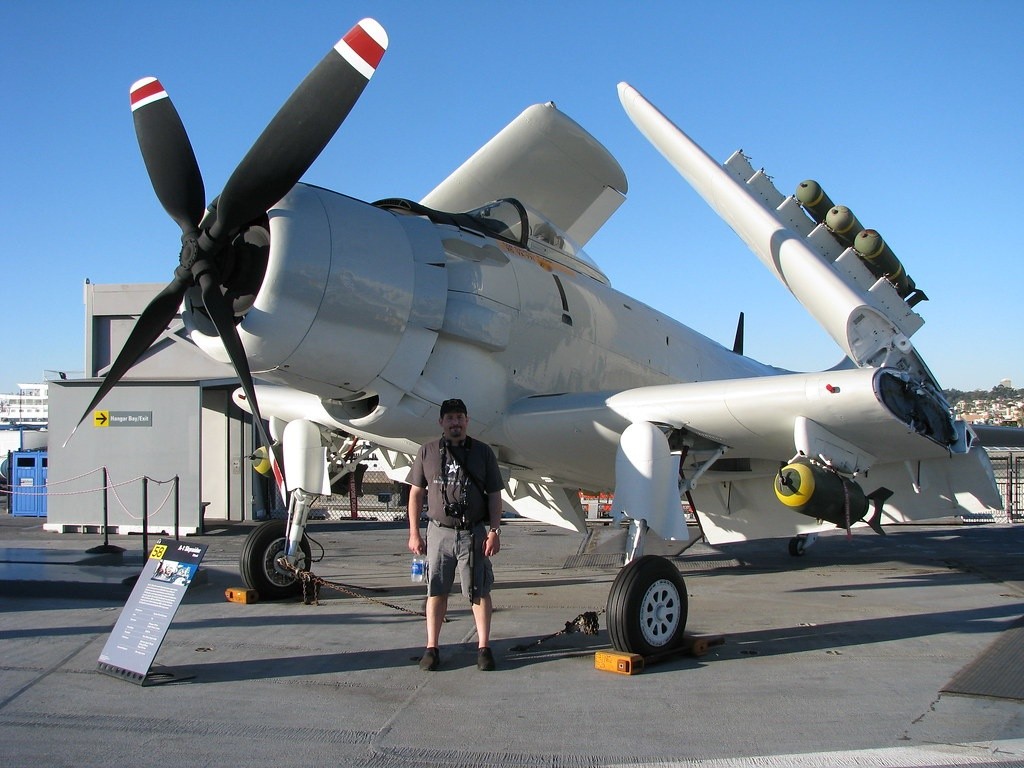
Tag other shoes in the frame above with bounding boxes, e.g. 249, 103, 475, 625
478, 646, 494, 671
419, 647, 440, 671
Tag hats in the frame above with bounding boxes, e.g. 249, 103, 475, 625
440, 398, 468, 418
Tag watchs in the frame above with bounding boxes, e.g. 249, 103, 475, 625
489, 527, 501, 535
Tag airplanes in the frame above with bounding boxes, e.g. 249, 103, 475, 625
59, 15, 1005, 660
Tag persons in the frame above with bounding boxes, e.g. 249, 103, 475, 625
404, 398, 504, 670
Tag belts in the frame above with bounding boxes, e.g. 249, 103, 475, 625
430, 518, 471, 530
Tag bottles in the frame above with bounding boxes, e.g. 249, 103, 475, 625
411, 555, 424, 582
